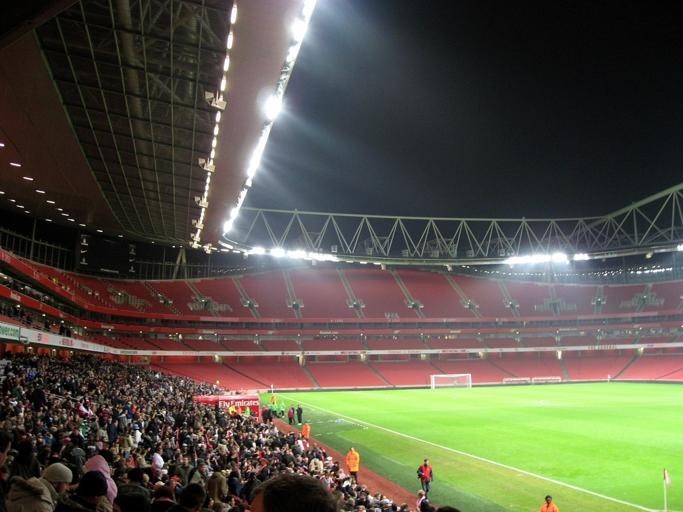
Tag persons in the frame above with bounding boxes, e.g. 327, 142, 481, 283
539, 495, 560, 512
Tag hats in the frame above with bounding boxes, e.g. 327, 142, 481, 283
76, 470, 108, 496
43, 462, 73, 483
